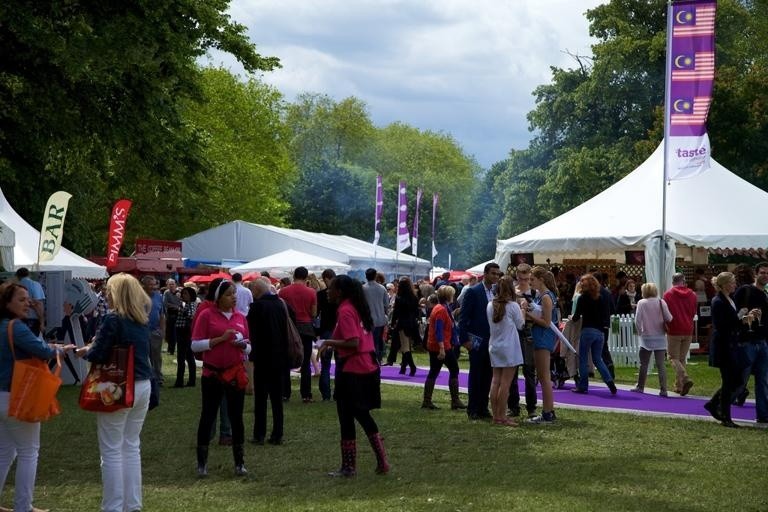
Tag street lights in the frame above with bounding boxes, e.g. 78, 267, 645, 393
278, 296, 305, 370
78, 313, 136, 416
8, 320, 63, 423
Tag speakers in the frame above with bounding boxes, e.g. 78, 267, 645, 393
448, 378, 468, 412
399, 351, 408, 374
366, 431, 391, 475
420, 378, 441, 413
228, 441, 248, 477
404, 350, 416, 376
195, 444, 212, 477
326, 438, 359, 480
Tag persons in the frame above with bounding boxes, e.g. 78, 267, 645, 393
74, 254, 768, 510
1, 282, 79, 510
15, 268, 47, 338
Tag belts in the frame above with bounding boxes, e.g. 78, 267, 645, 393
747, 308, 764, 332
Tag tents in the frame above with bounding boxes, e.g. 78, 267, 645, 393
0, 187, 107, 388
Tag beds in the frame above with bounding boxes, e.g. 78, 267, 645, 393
227, 247, 353, 275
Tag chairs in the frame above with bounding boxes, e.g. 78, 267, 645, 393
704, 387, 767, 427
571, 378, 698, 399
466, 405, 559, 427
